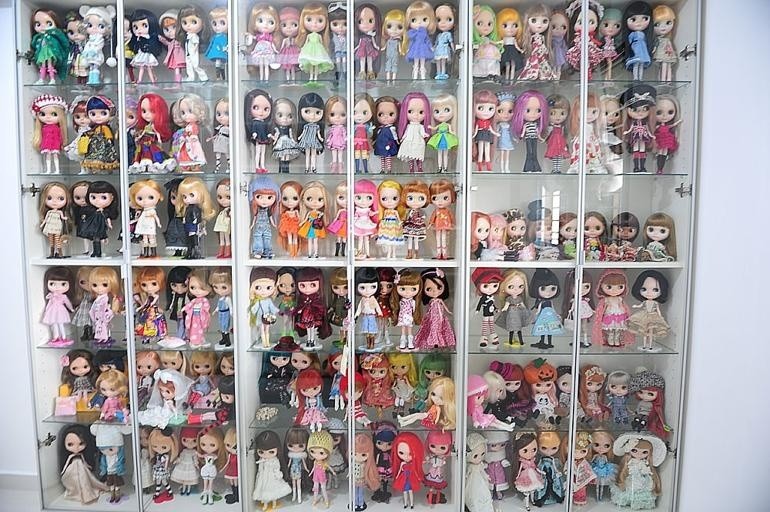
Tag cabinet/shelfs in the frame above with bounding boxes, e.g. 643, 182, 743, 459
18, 0, 700, 511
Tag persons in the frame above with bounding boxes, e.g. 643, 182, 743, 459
208, 266, 236, 349
193, 427, 227, 506
176, 4, 210, 83
148, 427, 180, 496
187, 375, 235, 437
126, 8, 163, 85
397, 91, 431, 175
136, 367, 197, 429
60, 8, 90, 84
508, 428, 547, 512
481, 431, 511, 501
133, 428, 154, 495
353, 179, 378, 258
259, 350, 294, 410
77, 4, 116, 83
611, 429, 668, 508
67, 181, 97, 257
424, 92, 461, 173
621, 0, 654, 82
338, 370, 376, 428
559, 428, 597, 506
431, 3, 458, 81
482, 370, 528, 429
210, 349, 235, 375
353, 92, 376, 173
268, 98, 301, 172
491, 91, 517, 174
391, 431, 426, 510
553, 365, 594, 425
473, 89, 501, 170
511, 89, 546, 172
375, 8, 406, 81
502, 209, 559, 262
37, 182, 72, 258
245, 89, 275, 172
171, 427, 200, 496
305, 430, 338, 510
252, 431, 294, 511
628, 370, 672, 439
348, 432, 380, 508
390, 269, 424, 350
32, 94, 70, 176
59, 424, 109, 502
561, 268, 598, 349
358, 354, 396, 419
91, 422, 132, 504
618, 83, 658, 173
283, 427, 314, 503
593, 8, 622, 81
249, 176, 282, 258
129, 92, 177, 172
205, 96, 231, 174
212, 177, 230, 255
275, 264, 298, 344
129, 181, 166, 259
326, 429, 350, 492
293, 268, 334, 347
40, 266, 75, 346
484, 214, 537, 262
495, 5, 527, 82
387, 351, 418, 416
594, 94, 626, 176
398, 179, 432, 258
86, 181, 120, 258
583, 213, 633, 262
375, 180, 404, 258
326, 349, 344, 411
284, 349, 321, 408
625, 269, 673, 351
602, 369, 635, 425
584, 427, 616, 501
650, 4, 677, 81
179, 349, 219, 409
176, 92, 212, 172
163, 178, 190, 257
465, 433, 497, 512
116, 183, 142, 255
593, 269, 636, 346
290, 369, 329, 431
495, 268, 532, 345
566, 0, 604, 81
640, 212, 678, 262
325, 181, 348, 256
323, 95, 347, 172
557, 211, 600, 261
133, 264, 168, 346
165, 266, 193, 341
155, 350, 192, 377
81, 94, 119, 174
298, 180, 332, 258
396, 375, 457, 434
246, 4, 281, 83
60, 349, 95, 408
157, 8, 186, 83
544, 8, 571, 79
372, 95, 400, 174
181, 268, 216, 349
471, 212, 520, 262
293, 4, 336, 82
401, 1, 438, 81
93, 369, 129, 422
27, 8, 74, 84
135, 351, 160, 409
414, 268, 456, 349
528, 268, 568, 349
112, 14, 138, 82
114, 95, 141, 172
274, 7, 301, 81
539, 93, 571, 174
578, 364, 612, 422
87, 266, 123, 347
533, 428, 568, 506
354, 266, 384, 349
323, 1, 348, 81
427, 178, 456, 258
86, 350, 127, 409
473, 267, 506, 346
407, 352, 449, 415
372, 422, 400, 503
521, 1, 557, 80
295, 92, 325, 174
326, 266, 351, 345
168, 97, 185, 171
275, 181, 307, 259
217, 428, 238, 503
203, 5, 231, 81
610, 212, 653, 262
377, 267, 398, 346
249, 268, 278, 347
354, 3, 382, 79
568, 92, 606, 174
523, 358, 563, 425
490, 360, 542, 420
419, 431, 453, 506
471, 5, 504, 83
61, 95, 95, 174
647, 94, 683, 174
467, 375, 516, 432
70, 266, 96, 342
527, 201, 570, 260
172, 175, 218, 257
